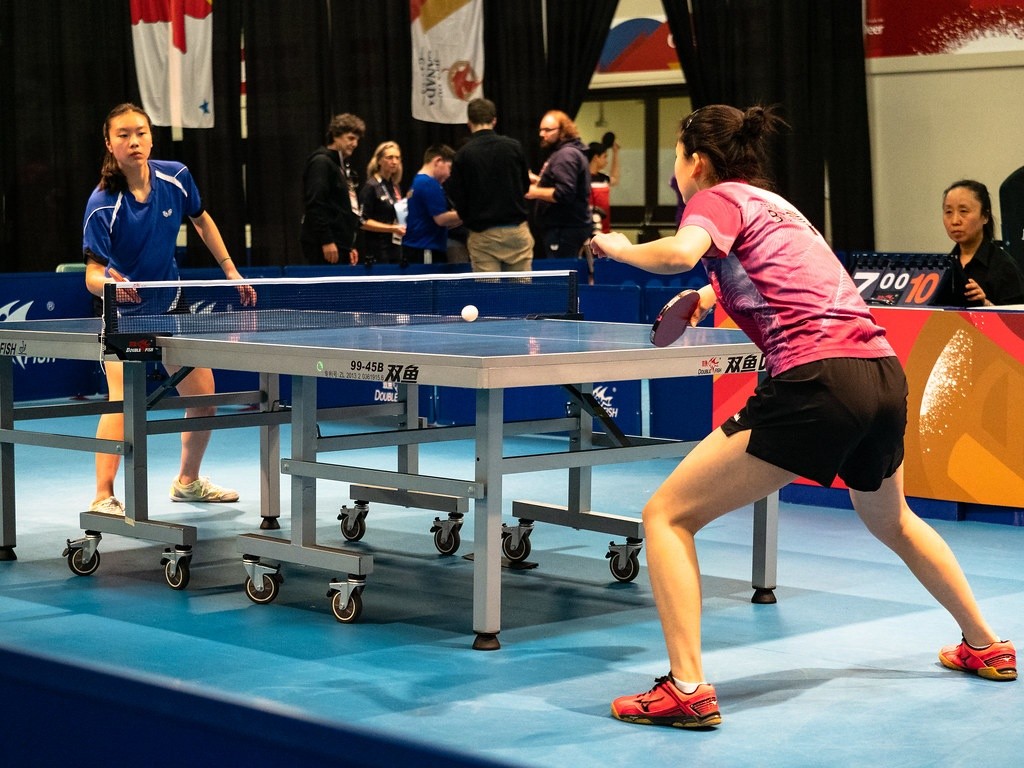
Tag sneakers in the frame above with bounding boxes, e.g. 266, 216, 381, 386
90, 496, 125, 516
938, 631, 1018, 679
170, 476, 240, 502
610, 671, 722, 727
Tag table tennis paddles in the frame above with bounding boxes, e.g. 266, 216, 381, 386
649, 288, 716, 348
108, 268, 141, 303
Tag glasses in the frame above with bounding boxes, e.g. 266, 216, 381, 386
538, 127, 559, 133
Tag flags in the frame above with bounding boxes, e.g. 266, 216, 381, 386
130, 0, 215, 129
409, 0, 485, 124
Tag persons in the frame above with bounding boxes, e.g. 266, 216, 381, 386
940, 179, 1023, 309
81, 103, 259, 521
584, 139, 621, 234
525, 110, 592, 260
445, 97, 535, 284
584, 102, 1018, 730
356, 139, 410, 265
298, 113, 366, 266
400, 142, 464, 264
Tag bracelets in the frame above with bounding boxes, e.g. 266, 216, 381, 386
219, 255, 232, 267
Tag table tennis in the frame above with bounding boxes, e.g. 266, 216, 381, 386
461, 304, 479, 323
